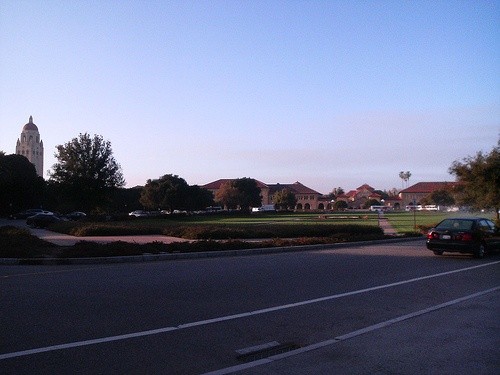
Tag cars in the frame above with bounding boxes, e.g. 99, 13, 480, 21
7, 205, 255, 221
370, 204, 488, 216
426, 217, 500, 259
